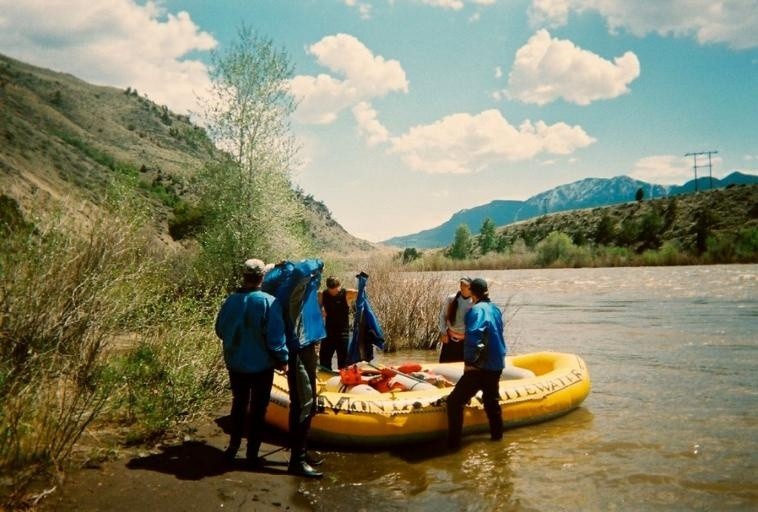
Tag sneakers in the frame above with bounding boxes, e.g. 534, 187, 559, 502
286, 460, 325, 478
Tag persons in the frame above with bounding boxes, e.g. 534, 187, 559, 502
435, 278, 508, 449
317, 270, 369, 374
262, 257, 330, 479
437, 276, 473, 363
212, 257, 290, 471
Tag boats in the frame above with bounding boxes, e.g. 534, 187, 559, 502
243, 347, 592, 451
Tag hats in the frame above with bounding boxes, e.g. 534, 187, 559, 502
241, 258, 265, 279
467, 277, 488, 291
458, 275, 472, 285
